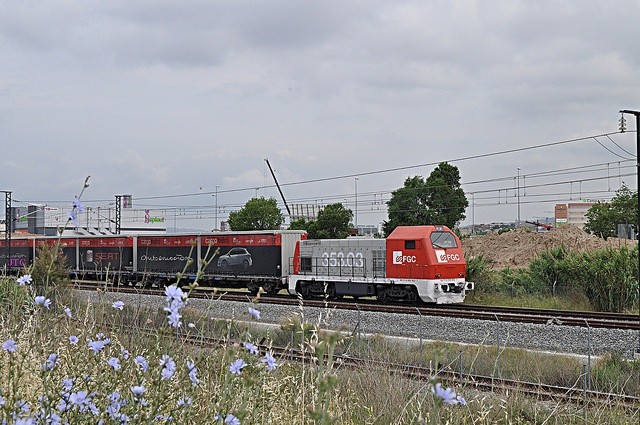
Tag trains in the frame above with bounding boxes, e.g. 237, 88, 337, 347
0, 225, 467, 306
